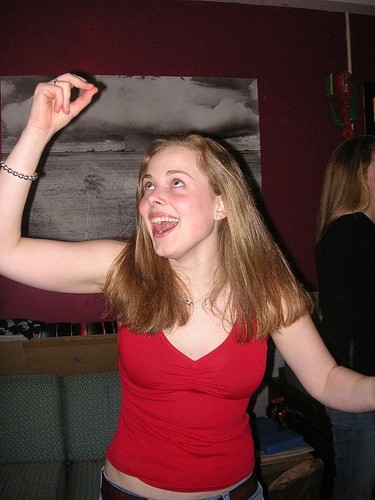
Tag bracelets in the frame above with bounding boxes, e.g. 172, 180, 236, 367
1, 159, 38, 182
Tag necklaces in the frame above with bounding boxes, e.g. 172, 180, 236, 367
183, 293, 208, 306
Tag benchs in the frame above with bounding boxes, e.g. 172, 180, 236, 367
0, 333, 122, 500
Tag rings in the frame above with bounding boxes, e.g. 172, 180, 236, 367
50, 79, 58, 85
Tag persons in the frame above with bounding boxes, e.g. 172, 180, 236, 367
0, 72, 375, 500
314, 136, 375, 500
